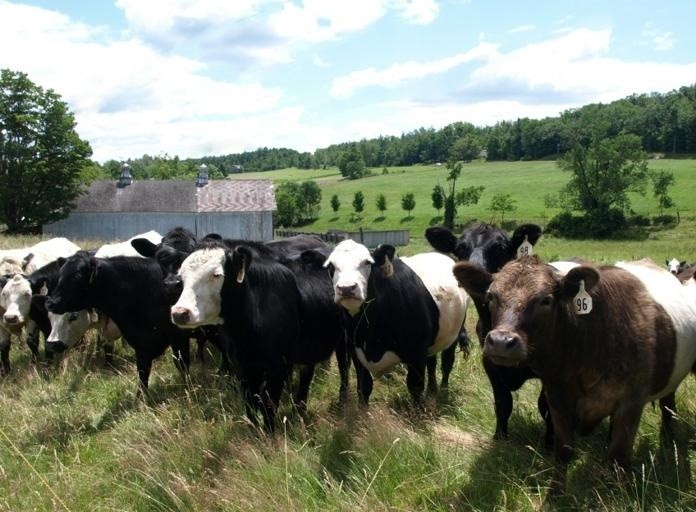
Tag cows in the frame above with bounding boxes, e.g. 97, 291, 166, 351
0, 220, 694, 478
